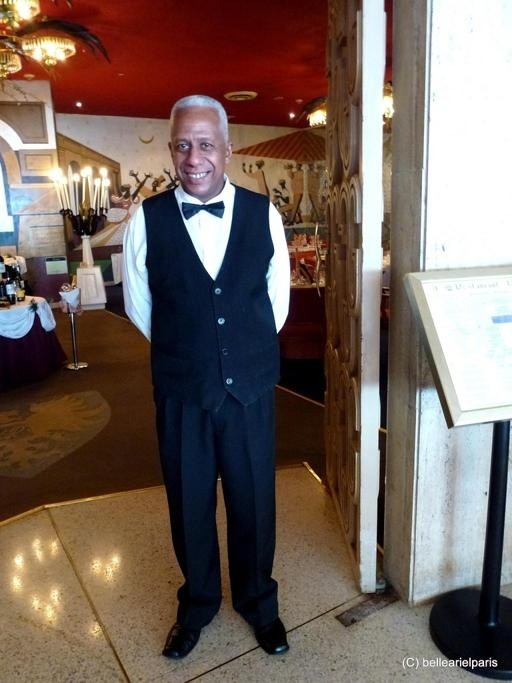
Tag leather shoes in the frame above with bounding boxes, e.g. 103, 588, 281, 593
163, 621, 205, 658
253, 616, 289, 656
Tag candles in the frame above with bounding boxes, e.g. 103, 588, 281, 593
50, 162, 112, 216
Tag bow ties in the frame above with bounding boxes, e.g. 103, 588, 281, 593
182, 200, 226, 220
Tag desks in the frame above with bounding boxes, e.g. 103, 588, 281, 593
0, 293, 67, 386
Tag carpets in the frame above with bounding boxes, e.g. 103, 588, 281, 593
0, 305, 386, 556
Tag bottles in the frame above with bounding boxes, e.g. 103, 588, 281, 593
6, 264, 25, 304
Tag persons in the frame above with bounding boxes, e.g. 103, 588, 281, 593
122, 94, 291, 659
